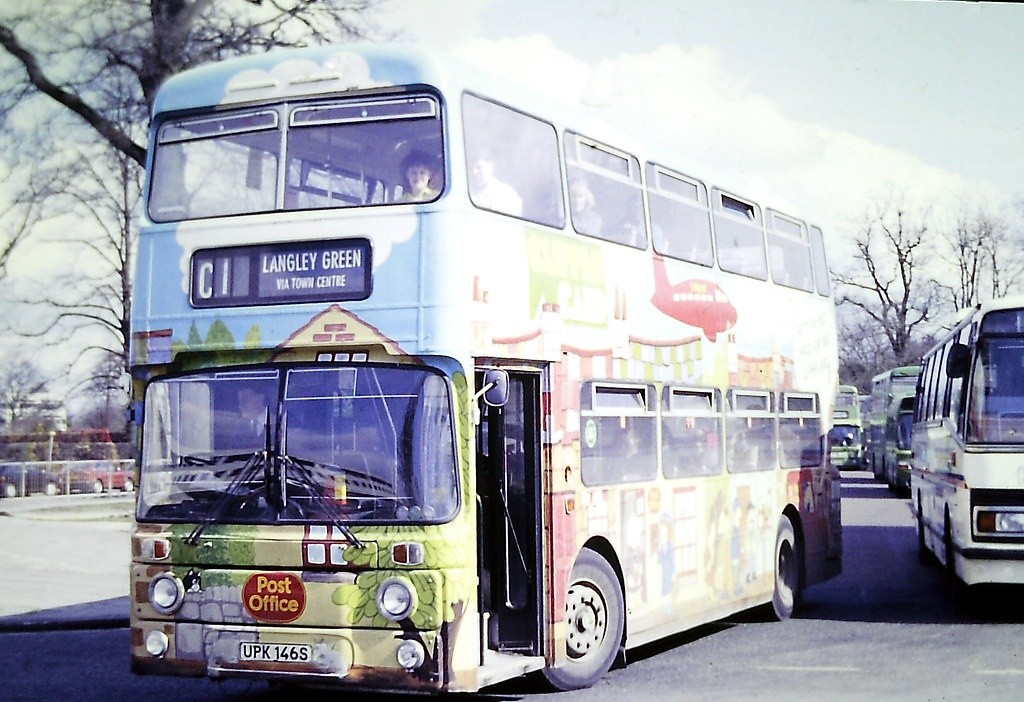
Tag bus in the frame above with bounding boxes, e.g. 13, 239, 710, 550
116, 37, 845, 695
907, 295, 1023, 599
867, 366, 930, 495
828, 385, 873, 471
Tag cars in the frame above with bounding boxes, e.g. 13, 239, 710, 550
60, 461, 136, 494
0, 464, 61, 498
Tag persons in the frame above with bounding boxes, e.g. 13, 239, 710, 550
398, 149, 665, 255
238, 387, 307, 466
611, 403, 817, 480
